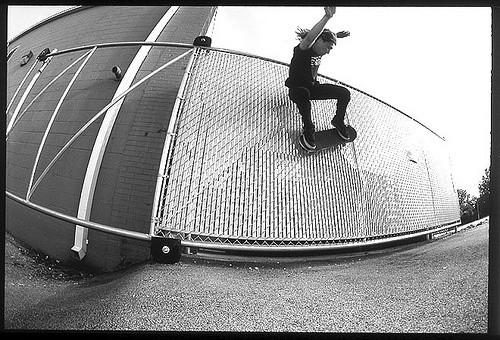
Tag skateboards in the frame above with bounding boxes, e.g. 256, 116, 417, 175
299, 125, 357, 153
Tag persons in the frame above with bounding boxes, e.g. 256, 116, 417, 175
285, 6, 351, 150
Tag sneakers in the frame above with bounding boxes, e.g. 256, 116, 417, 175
303, 121, 316, 150
331, 116, 350, 140
